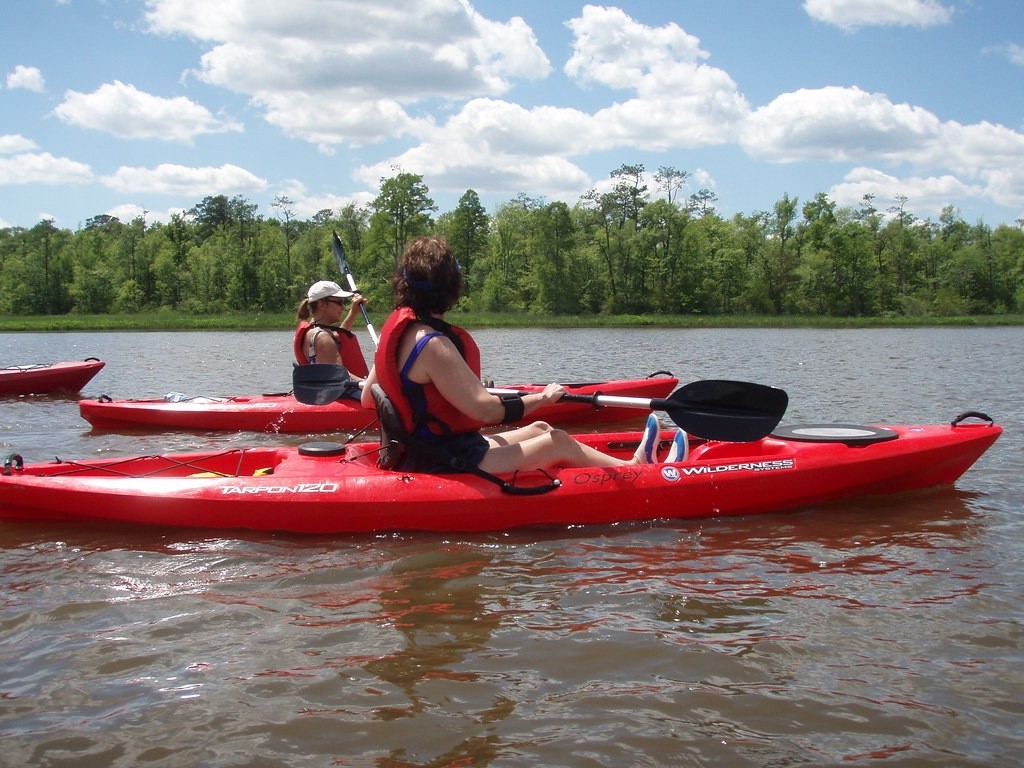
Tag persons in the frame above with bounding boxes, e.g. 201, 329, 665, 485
358, 235, 690, 473
294, 280, 371, 390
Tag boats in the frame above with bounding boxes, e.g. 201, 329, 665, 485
0, 357, 106, 397
0, 411, 1007, 539
75, 371, 682, 434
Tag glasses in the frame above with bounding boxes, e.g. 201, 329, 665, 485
323, 299, 343, 306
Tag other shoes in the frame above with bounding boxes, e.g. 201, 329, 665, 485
634, 413, 660, 464
487, 380, 494, 388
663, 427, 689, 463
482, 380, 488, 387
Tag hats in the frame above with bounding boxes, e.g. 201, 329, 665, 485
306, 280, 354, 303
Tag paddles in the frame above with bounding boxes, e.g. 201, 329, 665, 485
483, 379, 788, 443
331, 229, 378, 346
